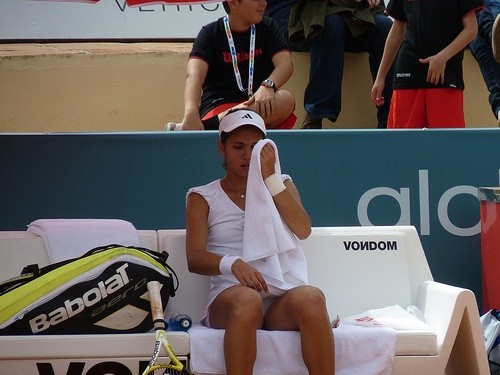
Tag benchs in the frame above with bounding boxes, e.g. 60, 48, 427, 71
0, 225, 491, 375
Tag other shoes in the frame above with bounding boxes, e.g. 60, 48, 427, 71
300, 113, 323, 129
164, 121, 182, 131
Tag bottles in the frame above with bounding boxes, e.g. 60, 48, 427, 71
168, 312, 192, 332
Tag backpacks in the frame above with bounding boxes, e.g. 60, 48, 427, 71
480, 309, 500, 375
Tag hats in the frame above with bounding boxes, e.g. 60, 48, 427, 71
218, 110, 267, 138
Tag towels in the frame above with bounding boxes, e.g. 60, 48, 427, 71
28, 218, 140, 265
242, 138, 309, 289
188, 322, 397, 374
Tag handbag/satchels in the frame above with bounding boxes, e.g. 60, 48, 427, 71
0, 244, 178, 336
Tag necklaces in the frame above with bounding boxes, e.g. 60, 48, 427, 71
224, 177, 246, 198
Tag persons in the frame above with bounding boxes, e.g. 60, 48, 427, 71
264, 0, 500, 129
166, 0, 295, 130
185, 108, 335, 375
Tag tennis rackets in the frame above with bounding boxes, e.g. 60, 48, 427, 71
142, 281, 192, 375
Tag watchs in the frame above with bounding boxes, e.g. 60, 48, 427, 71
263, 80, 277, 91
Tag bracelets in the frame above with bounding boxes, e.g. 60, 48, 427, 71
265, 173, 287, 197
261, 83, 269, 88
219, 255, 244, 276
